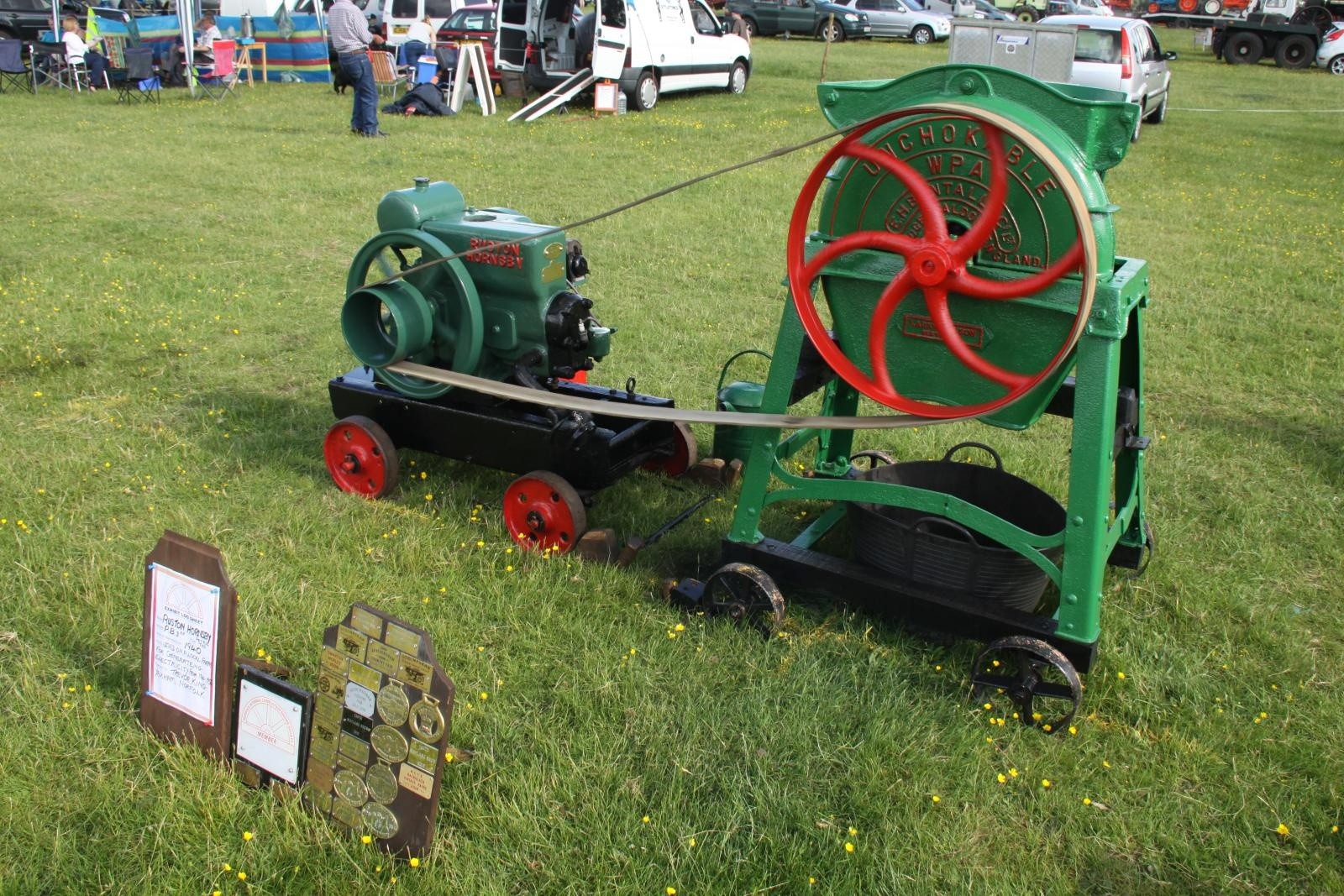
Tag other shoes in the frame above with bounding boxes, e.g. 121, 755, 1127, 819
362, 131, 390, 138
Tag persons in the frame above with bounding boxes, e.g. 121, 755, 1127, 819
730, 7, 753, 47
328, 0, 391, 138
575, 5, 596, 68
367, 13, 382, 36
30, 16, 65, 85
62, 14, 114, 93
404, 14, 437, 76
154, 14, 222, 88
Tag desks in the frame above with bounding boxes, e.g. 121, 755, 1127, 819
237, 41, 266, 82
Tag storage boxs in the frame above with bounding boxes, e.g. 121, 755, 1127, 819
417, 55, 439, 83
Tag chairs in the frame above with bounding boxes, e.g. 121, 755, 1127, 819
1, 31, 253, 106
365, 50, 415, 98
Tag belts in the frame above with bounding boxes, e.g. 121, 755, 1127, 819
341, 48, 365, 56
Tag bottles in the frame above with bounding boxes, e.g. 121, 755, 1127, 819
227, 25, 235, 39
242, 13, 255, 39
617, 88, 626, 115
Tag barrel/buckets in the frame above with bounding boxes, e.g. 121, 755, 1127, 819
849, 442, 1066, 615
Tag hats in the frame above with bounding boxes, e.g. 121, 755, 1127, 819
367, 13, 377, 20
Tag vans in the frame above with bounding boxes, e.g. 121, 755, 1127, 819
493, 0, 755, 109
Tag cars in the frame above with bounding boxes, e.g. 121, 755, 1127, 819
1316, 22, 1344, 75
289, 0, 509, 98
827, 0, 953, 45
936, 0, 1115, 27
1032, 13, 1179, 143
723, 0, 872, 43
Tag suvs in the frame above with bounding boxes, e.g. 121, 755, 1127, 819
0, 0, 133, 53
